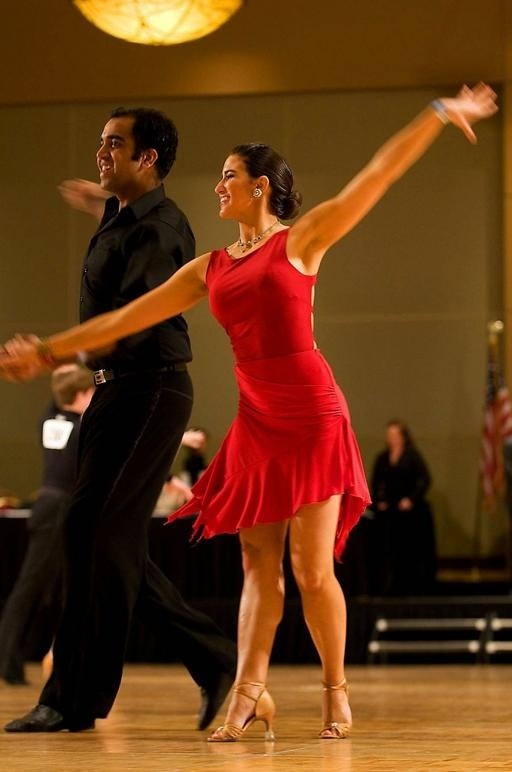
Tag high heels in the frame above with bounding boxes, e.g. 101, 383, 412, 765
210, 675, 275, 742
317, 676, 351, 739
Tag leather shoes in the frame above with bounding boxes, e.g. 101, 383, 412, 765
198, 663, 234, 730
3, 703, 95, 733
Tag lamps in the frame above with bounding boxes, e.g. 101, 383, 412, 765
70, 0, 249, 51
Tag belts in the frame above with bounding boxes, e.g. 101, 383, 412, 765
92, 364, 188, 382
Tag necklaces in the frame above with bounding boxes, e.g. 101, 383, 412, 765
236, 220, 279, 254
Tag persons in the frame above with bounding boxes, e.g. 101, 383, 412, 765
366, 418, 442, 599
0, 365, 206, 688
0, 79, 500, 744
1, 107, 238, 734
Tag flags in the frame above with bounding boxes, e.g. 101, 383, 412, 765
476, 335, 512, 513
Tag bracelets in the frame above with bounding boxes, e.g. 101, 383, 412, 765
37, 336, 54, 364
427, 98, 450, 126
